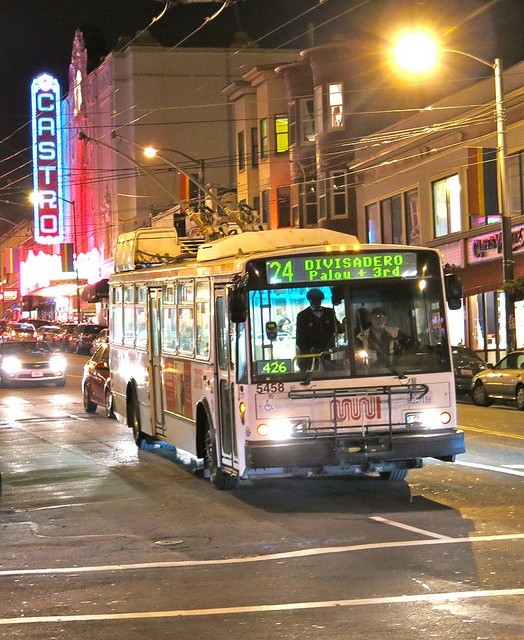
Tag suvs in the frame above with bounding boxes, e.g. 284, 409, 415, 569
52, 323, 78, 350
62, 324, 108, 353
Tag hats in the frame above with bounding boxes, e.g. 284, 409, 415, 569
371, 307, 386, 314
307, 289, 324, 300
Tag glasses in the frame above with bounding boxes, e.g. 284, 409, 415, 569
374, 315, 386, 320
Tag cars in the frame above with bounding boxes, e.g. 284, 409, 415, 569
1, 321, 36, 340
36, 326, 59, 344
89, 329, 109, 357
0, 340, 66, 387
451, 346, 493, 394
81, 344, 114, 418
470, 351, 524, 411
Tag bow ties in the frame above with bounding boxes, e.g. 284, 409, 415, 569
313, 309, 323, 312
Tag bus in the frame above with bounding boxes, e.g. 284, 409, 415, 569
107, 228, 466, 490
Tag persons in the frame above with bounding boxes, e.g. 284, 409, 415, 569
354, 306, 415, 363
294, 288, 345, 365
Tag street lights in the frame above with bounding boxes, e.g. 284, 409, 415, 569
29, 193, 80, 325
390, 29, 517, 368
143, 145, 205, 227
